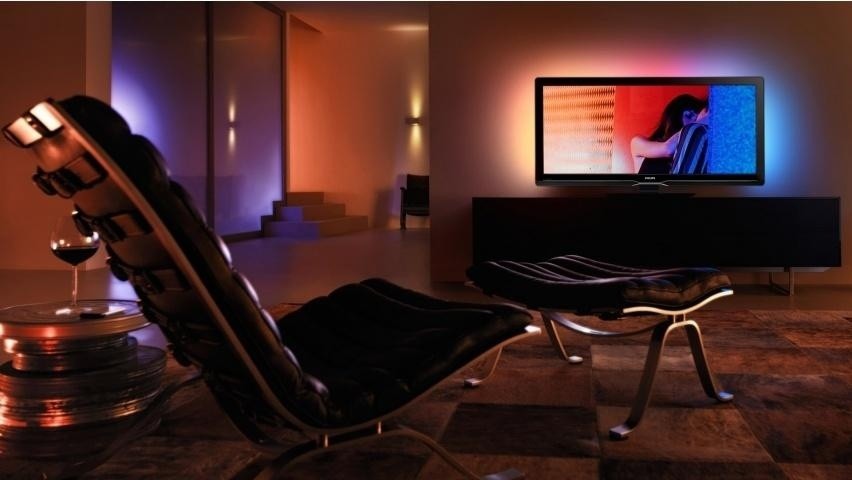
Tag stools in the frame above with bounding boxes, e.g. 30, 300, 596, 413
463, 254, 735, 437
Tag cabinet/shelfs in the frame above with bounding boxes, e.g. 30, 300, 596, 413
471, 196, 842, 297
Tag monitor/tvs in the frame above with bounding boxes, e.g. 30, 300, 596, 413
535, 76, 764, 190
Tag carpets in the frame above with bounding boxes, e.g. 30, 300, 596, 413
1, 302, 852, 480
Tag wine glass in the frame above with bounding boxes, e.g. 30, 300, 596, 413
48, 211, 100, 315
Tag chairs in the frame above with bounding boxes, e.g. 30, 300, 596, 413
1, 93, 542, 479
400, 174, 429, 232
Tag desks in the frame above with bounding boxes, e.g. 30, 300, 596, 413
0, 299, 166, 462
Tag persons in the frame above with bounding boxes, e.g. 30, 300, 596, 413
630, 94, 709, 174
